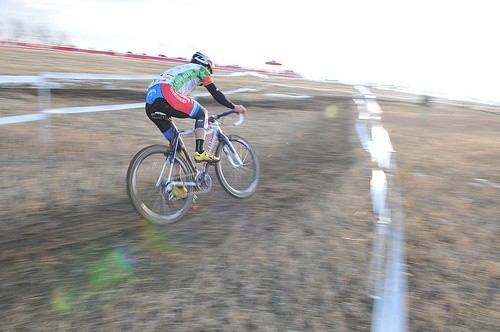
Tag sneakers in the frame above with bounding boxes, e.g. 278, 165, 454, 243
194, 150, 221, 163
173, 186, 196, 200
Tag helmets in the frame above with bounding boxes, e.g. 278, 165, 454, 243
191, 51, 215, 74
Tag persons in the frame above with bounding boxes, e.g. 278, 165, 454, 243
145, 51, 247, 205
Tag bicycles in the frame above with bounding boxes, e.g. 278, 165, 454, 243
125, 110, 259, 226
